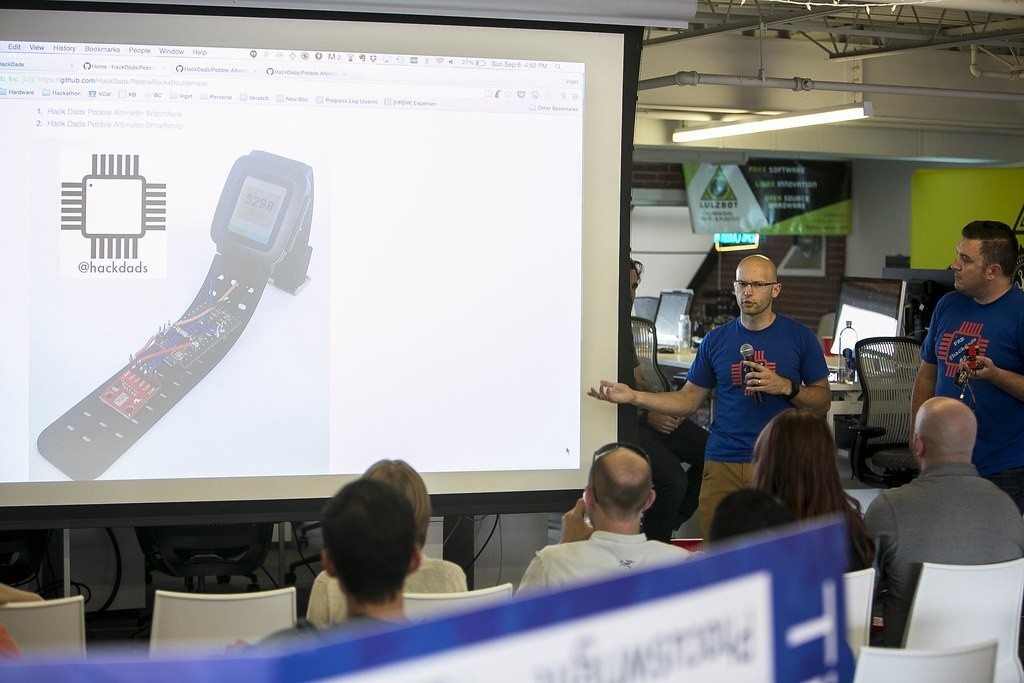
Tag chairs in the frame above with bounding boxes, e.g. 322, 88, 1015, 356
0, 595, 86, 663
403, 583, 513, 626
844, 557, 1024, 683
846, 336, 919, 489
146, 587, 296, 661
629, 316, 671, 393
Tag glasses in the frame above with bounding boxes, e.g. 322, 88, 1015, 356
593, 443, 650, 502
734, 280, 777, 292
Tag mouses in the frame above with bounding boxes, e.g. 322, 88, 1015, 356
659, 348, 675, 354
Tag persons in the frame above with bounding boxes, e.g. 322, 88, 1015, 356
512, 446, 704, 603
885, 219, 1024, 516
707, 408, 875, 576
863, 396, 1024, 650
228, 480, 423, 663
584, 254, 832, 540
305, 446, 467, 625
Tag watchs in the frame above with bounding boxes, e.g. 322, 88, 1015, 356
638, 408, 648, 426
37, 150, 316, 481
782, 379, 801, 401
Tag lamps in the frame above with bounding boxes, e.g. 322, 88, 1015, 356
671, 11, 875, 143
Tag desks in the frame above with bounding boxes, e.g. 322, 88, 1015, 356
635, 344, 919, 444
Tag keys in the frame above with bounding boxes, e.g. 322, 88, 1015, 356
959, 378, 976, 404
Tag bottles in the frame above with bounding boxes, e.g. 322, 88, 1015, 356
839, 320, 859, 384
676, 313, 692, 355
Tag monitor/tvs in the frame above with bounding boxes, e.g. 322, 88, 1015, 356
654, 289, 693, 348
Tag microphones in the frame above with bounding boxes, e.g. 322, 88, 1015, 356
740, 343, 766, 404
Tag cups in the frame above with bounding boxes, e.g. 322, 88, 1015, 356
822, 339, 835, 357
669, 538, 705, 553
